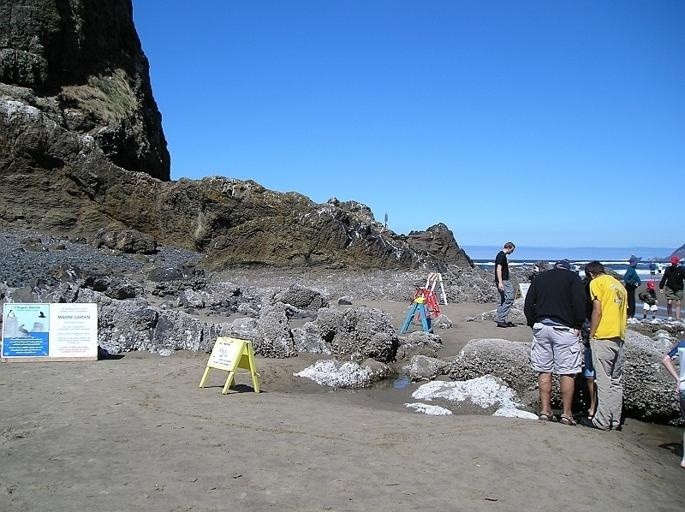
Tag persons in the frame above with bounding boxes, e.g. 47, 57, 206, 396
580, 312, 599, 420
649, 260, 657, 281
659, 256, 685, 321
579, 260, 628, 431
524, 260, 589, 426
622, 255, 642, 325
639, 281, 659, 323
658, 262, 664, 278
492, 242, 518, 327
661, 331, 685, 468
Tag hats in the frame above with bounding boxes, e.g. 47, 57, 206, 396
647, 281, 654, 289
627, 255, 642, 262
671, 256, 679, 265
555, 260, 571, 270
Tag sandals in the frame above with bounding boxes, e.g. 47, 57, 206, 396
560, 413, 577, 426
539, 410, 559, 422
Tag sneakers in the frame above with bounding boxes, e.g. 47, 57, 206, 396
493, 321, 517, 328
578, 416, 596, 428
